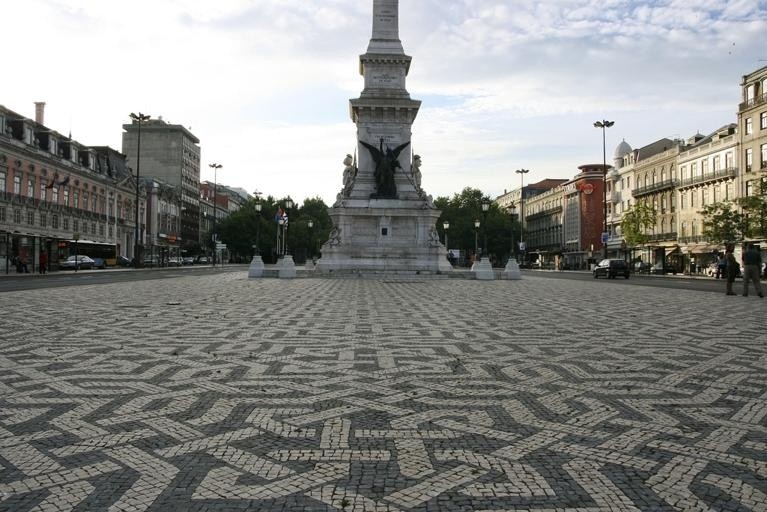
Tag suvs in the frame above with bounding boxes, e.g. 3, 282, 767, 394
593, 258, 631, 282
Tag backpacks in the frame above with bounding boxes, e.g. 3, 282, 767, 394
718, 258, 727, 268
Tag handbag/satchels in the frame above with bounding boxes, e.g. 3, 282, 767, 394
735, 263, 740, 275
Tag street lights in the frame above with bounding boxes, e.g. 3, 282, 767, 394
207, 162, 223, 267
441, 220, 450, 259
506, 203, 521, 281
277, 215, 288, 267
592, 119, 618, 259
280, 196, 298, 278
515, 168, 530, 261
471, 218, 482, 272
128, 112, 151, 271
476, 196, 496, 281
306, 218, 315, 270
249, 200, 267, 278
252, 190, 264, 253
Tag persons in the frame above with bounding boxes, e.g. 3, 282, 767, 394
741, 243, 764, 298
723, 243, 739, 296
375, 137, 395, 200
38, 250, 48, 275
714, 254, 726, 279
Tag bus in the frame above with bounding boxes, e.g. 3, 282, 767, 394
58, 239, 118, 270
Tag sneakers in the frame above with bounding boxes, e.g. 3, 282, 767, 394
726, 291, 765, 298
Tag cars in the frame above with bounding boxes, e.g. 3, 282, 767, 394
59, 255, 97, 272
635, 260, 683, 276
143, 254, 215, 268
707, 259, 741, 280
116, 256, 130, 268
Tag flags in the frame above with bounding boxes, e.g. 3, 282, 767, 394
271, 207, 288, 230
